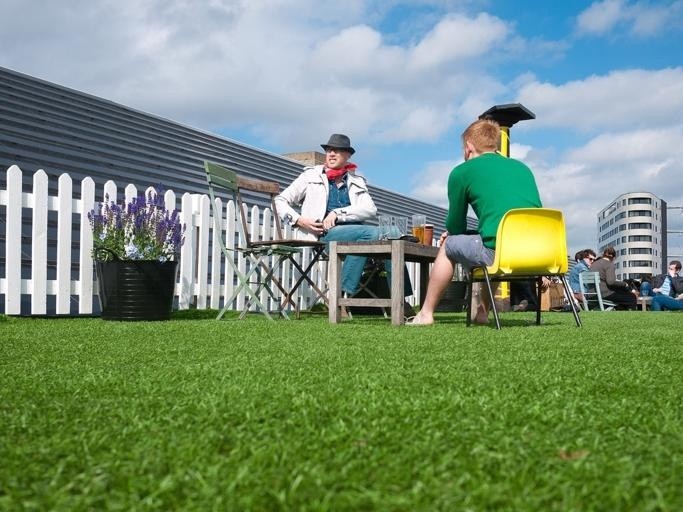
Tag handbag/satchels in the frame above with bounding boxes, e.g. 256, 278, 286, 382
541, 279, 565, 312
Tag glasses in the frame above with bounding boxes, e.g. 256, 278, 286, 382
586, 256, 595, 261
325, 148, 348, 153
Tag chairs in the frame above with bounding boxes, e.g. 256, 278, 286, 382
235, 177, 329, 319
578, 271, 615, 311
203, 159, 302, 323
467, 207, 583, 330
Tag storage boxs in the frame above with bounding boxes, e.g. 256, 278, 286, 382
540, 283, 564, 311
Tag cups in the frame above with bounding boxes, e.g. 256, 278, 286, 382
379, 214, 434, 246
642, 290, 648, 297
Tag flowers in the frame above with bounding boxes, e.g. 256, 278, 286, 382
87, 183, 185, 261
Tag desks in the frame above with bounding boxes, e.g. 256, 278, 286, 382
636, 297, 654, 311
329, 241, 440, 327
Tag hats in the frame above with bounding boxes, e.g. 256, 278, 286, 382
321, 134, 355, 155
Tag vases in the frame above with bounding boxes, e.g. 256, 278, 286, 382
435, 281, 465, 311
96, 262, 177, 321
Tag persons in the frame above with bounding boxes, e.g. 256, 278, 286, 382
404, 118, 542, 325
569, 247, 683, 311
511, 277, 549, 312
271, 134, 416, 322
